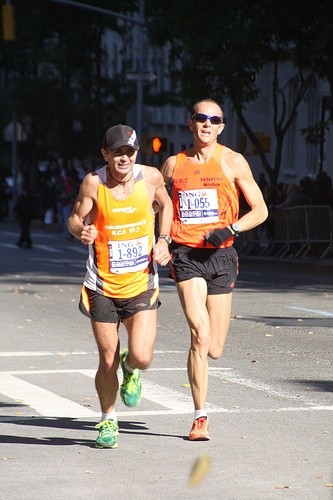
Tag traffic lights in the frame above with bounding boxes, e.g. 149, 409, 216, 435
150, 136, 167, 153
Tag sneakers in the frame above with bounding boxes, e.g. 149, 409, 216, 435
119, 347, 143, 408
94, 415, 119, 449
187, 410, 211, 441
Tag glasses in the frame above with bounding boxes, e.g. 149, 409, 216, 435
192, 113, 222, 125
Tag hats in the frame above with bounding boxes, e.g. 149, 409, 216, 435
102, 124, 139, 152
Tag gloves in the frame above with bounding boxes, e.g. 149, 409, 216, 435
204, 224, 236, 247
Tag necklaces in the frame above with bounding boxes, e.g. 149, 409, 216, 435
108, 167, 133, 187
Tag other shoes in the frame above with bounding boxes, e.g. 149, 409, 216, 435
15, 237, 32, 249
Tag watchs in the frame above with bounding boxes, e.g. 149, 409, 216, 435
230, 223, 240, 239
157, 234, 171, 244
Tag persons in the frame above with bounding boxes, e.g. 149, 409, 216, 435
152, 99, 268, 441
257, 170, 333, 258
0, 152, 82, 250
66, 123, 174, 449
